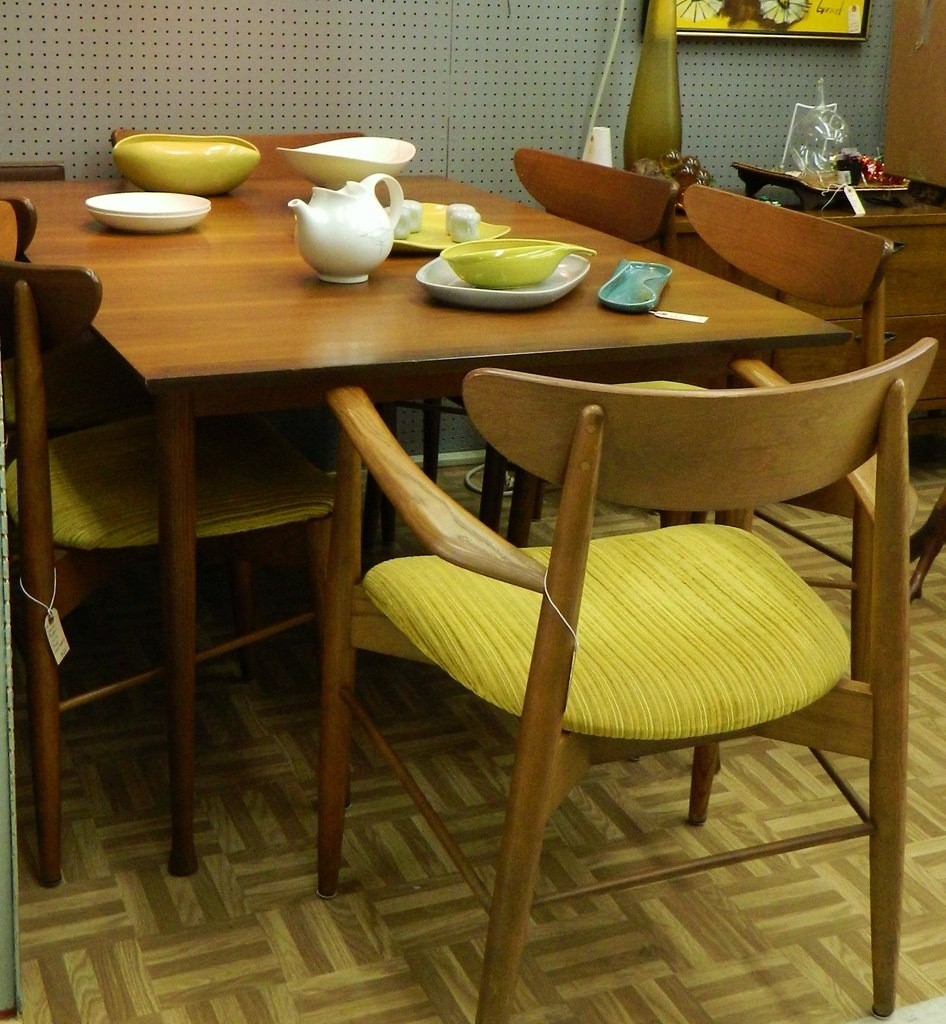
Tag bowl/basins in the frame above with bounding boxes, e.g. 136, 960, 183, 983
274, 135, 419, 193
438, 238, 598, 289
110, 134, 261, 197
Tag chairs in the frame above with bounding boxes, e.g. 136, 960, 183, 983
0, 191, 335, 888
321, 336, 942, 1022
416, 150, 680, 533
504, 181, 892, 555
112, 128, 391, 556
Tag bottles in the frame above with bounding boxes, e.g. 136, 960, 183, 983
622, 0, 683, 170
585, 125, 615, 169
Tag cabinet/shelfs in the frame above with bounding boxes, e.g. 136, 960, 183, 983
661, 188, 946, 413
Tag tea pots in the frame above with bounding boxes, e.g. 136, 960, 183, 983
287, 172, 404, 284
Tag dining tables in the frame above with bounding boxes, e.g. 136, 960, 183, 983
1, 176, 853, 873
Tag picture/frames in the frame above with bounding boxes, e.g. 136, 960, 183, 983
644, 1, 871, 41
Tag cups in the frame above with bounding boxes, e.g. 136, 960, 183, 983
402, 199, 422, 233
450, 210, 480, 242
385, 206, 410, 240
445, 204, 475, 235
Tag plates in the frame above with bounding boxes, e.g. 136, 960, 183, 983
596, 260, 673, 314
84, 191, 212, 214
414, 253, 590, 310
83, 207, 211, 236
383, 201, 513, 254
